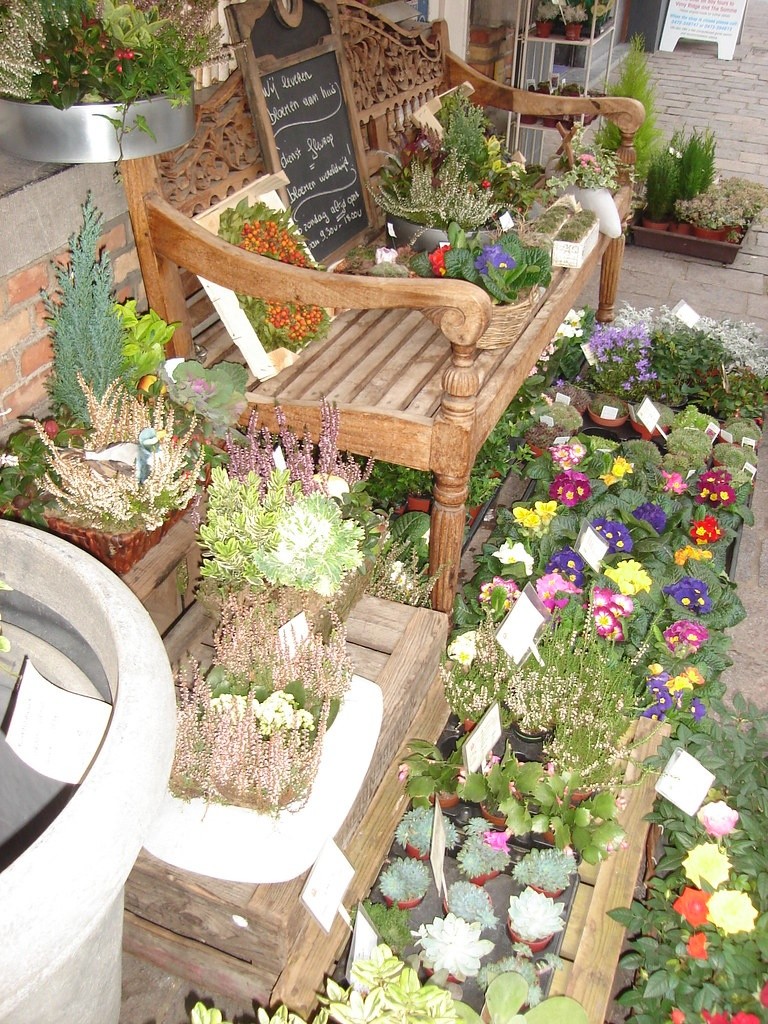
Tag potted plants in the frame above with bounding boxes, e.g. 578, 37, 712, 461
525, 422, 564, 457
524, 193, 600, 268
548, 402, 582, 435
189, 806, 578, 1024
345, 376, 546, 526
641, 122, 721, 236
587, 394, 763, 470
521, 81, 606, 130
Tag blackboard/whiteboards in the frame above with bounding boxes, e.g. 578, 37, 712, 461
246, 36, 380, 268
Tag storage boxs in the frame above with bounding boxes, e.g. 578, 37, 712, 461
630, 205, 754, 265
546, 715, 672, 1024
123, 591, 453, 1024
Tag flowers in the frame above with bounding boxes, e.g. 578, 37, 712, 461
546, 121, 639, 189
674, 176, 768, 243
400, 436, 755, 866
528, 299, 768, 425
367, 85, 544, 246
333, 221, 553, 304
535, 1, 588, 24
0, 189, 250, 528
547, 379, 591, 411
605, 691, 767, 1023
0, 0, 250, 186
168, 392, 395, 823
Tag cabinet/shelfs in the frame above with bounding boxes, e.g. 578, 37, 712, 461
506, 0, 618, 167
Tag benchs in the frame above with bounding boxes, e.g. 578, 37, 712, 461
119, 0, 645, 638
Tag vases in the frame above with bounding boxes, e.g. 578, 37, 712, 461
745, 215, 755, 229
571, 787, 591, 803
695, 227, 725, 241
515, 730, 543, 743
0, 436, 229, 576
546, 829, 575, 849
312, 543, 385, 645
0, 82, 196, 164
431, 789, 460, 806
474, 281, 542, 348
385, 212, 498, 255
565, 22, 582, 41
464, 718, 475, 730
575, 404, 587, 415
726, 224, 743, 242
536, 20, 553, 38
665, 395, 688, 407
581, 427, 621, 444
479, 802, 508, 826
